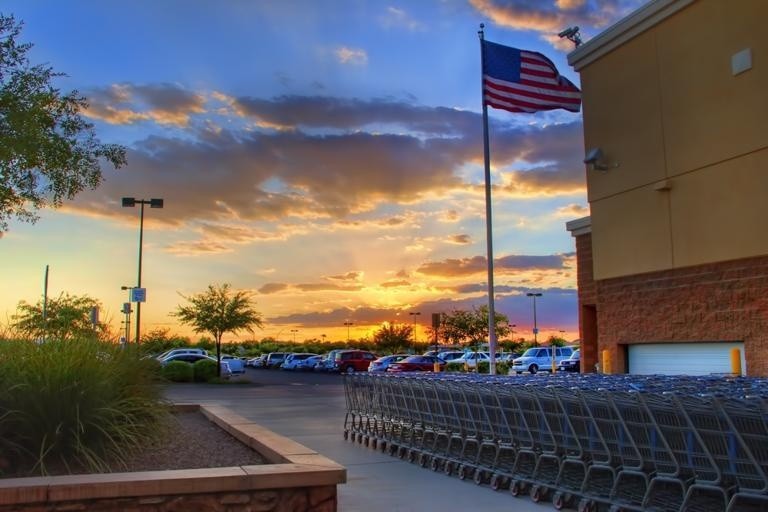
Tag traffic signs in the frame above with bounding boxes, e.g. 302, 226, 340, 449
131, 287, 145, 302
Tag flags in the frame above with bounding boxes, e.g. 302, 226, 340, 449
482, 38, 584, 115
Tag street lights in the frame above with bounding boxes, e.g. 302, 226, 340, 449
120, 286, 136, 358
409, 312, 420, 355
526, 293, 541, 347
343, 322, 353, 341
122, 196, 163, 357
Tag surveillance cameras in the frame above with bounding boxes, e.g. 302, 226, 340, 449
557, 25, 580, 39
581, 146, 602, 166
